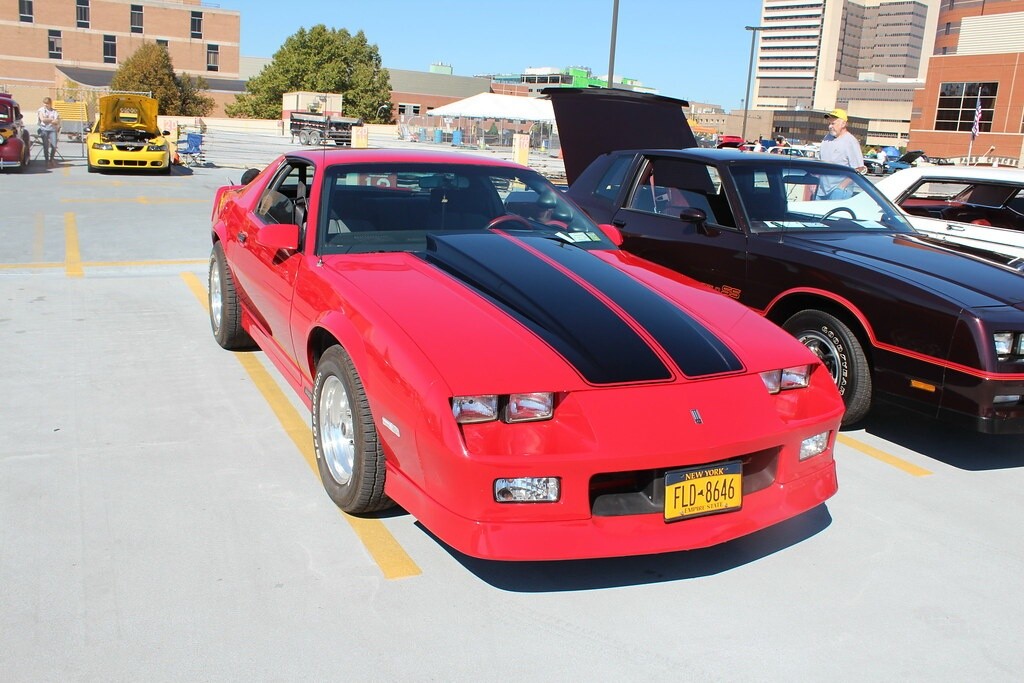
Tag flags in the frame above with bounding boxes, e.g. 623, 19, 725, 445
972, 86, 983, 137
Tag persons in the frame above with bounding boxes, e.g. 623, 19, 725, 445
472, 121, 480, 145
750, 135, 788, 155
808, 109, 867, 201
866, 144, 890, 171
38, 97, 60, 166
489, 122, 498, 144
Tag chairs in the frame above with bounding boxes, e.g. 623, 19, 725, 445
30, 128, 64, 162
754, 193, 786, 221
966, 208, 1020, 230
428, 179, 497, 231
325, 190, 377, 234
176, 133, 206, 170
662, 187, 708, 221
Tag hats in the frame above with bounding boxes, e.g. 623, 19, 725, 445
823, 109, 848, 121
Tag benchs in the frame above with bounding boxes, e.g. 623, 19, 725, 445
360, 195, 444, 230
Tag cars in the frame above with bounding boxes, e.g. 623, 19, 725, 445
82, 93, 173, 174
203, 145, 849, 566
0, 91, 33, 171
502, 86, 1024, 447
787, 161, 1024, 272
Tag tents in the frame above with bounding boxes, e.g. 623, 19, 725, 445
425, 92, 557, 148
